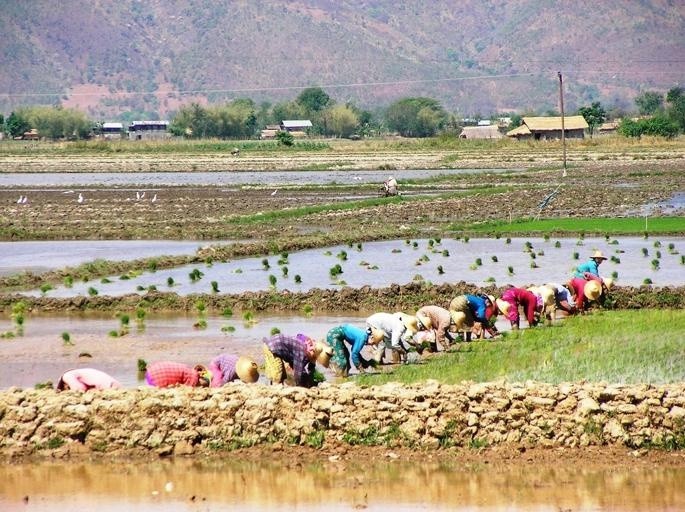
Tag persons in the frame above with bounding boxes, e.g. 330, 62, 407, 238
574, 251, 608, 274
58, 369, 118, 390
387, 176, 398, 196
501, 286, 547, 330
206, 353, 257, 384
366, 311, 418, 362
391, 311, 431, 361
478, 298, 513, 338
262, 330, 318, 385
528, 285, 555, 321
143, 361, 213, 385
450, 293, 498, 341
299, 333, 337, 384
326, 323, 385, 376
417, 306, 468, 350
548, 270, 612, 315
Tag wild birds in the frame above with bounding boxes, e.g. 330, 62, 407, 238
77, 192, 84, 204
150, 193, 157, 203
142, 191, 146, 199
135, 191, 141, 200
270, 189, 278, 197
16, 195, 28, 204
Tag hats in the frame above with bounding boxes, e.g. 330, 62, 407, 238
450, 309, 466, 329
308, 337, 336, 368
235, 355, 259, 383
583, 251, 613, 301
401, 312, 431, 333
482, 292, 511, 317
369, 325, 385, 349
533, 287, 555, 306
194, 363, 213, 388
